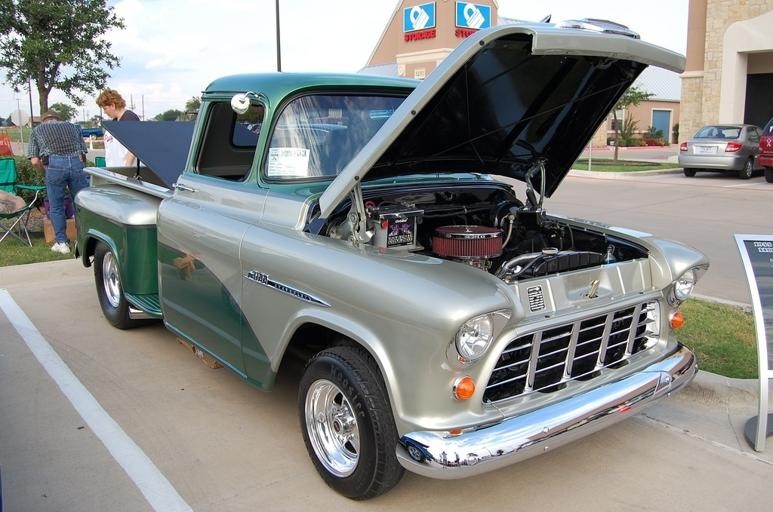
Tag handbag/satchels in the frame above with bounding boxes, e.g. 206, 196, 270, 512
44, 197, 73, 220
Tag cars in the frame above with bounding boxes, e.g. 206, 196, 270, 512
676, 121, 766, 180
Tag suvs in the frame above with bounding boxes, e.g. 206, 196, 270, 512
753, 116, 772, 185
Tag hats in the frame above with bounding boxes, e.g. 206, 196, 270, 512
41, 111, 59, 122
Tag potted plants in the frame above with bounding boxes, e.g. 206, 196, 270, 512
645, 127, 665, 146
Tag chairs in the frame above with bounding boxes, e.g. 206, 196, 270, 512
0, 158, 46, 247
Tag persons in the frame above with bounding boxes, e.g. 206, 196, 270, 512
27, 107, 89, 254
94, 87, 146, 168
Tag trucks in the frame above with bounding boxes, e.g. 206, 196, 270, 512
74, 124, 104, 142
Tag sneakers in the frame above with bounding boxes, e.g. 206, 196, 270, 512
52, 241, 69, 254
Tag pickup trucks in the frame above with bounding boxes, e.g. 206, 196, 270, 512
69, 13, 714, 504
